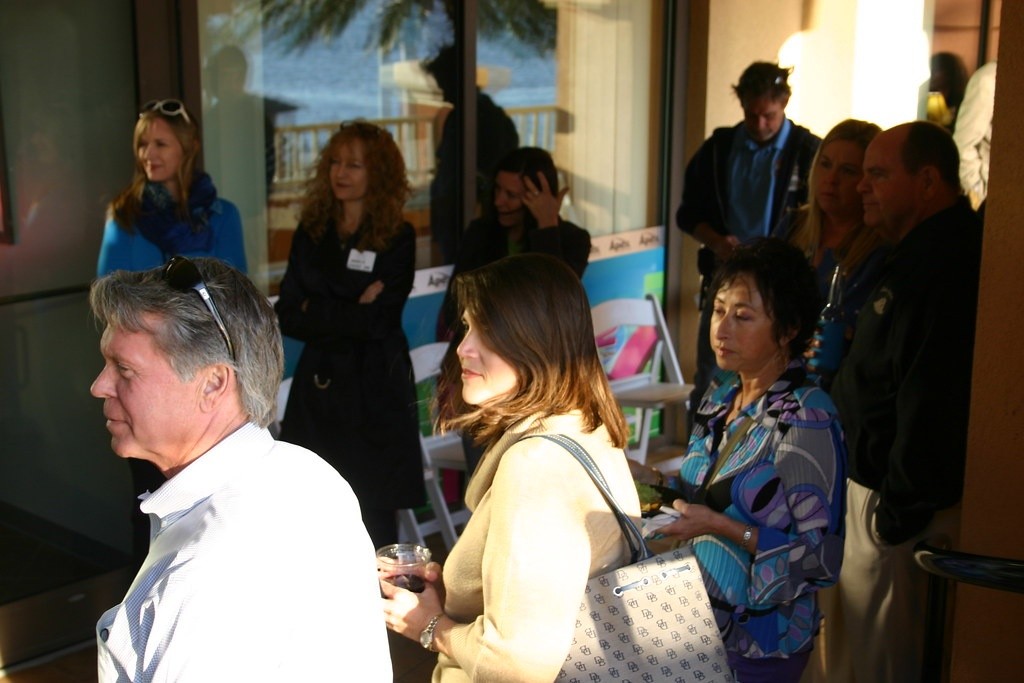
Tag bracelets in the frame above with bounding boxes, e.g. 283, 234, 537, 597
741, 524, 752, 550
652, 468, 663, 486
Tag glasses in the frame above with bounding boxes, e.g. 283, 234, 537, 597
162, 256, 237, 378
138, 99, 191, 124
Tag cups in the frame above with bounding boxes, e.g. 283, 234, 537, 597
378, 543, 432, 602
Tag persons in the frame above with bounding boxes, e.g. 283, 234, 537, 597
433, 147, 591, 507
272, 122, 428, 550
931, 51, 967, 129
675, 62, 835, 443
382, 255, 642, 683
421, 45, 518, 265
775, 120, 982, 683
628, 239, 847, 683
91, 260, 393, 683
93, 108, 248, 567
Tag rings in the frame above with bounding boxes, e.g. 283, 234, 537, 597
534, 190, 539, 196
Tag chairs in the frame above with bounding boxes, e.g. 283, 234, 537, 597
396, 343, 475, 556
588, 292, 699, 474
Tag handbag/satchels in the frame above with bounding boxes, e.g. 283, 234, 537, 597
518, 431, 735, 683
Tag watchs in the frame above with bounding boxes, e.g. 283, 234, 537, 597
419, 613, 446, 652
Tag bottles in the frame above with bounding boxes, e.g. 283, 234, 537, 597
810, 264, 849, 378
